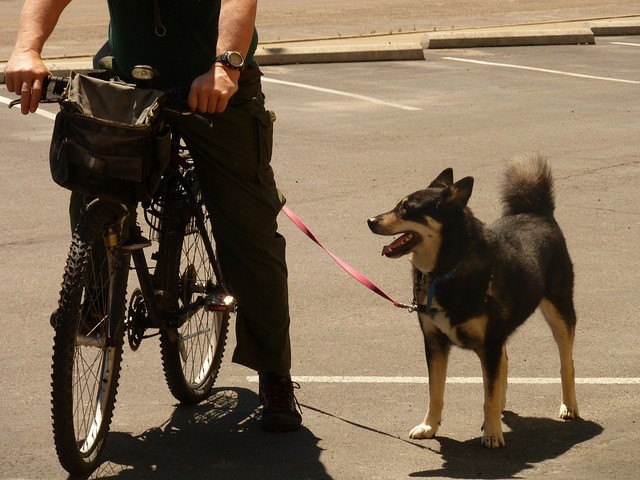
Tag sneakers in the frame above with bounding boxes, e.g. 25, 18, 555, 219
257, 370, 305, 432
50, 305, 99, 338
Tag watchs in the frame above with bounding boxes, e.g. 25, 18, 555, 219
215, 52, 245, 74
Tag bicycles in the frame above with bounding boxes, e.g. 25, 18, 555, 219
4, 75, 236, 476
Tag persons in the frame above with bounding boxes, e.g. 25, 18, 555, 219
6, 0, 302, 432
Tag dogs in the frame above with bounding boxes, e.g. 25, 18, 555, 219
367, 154, 579, 450
